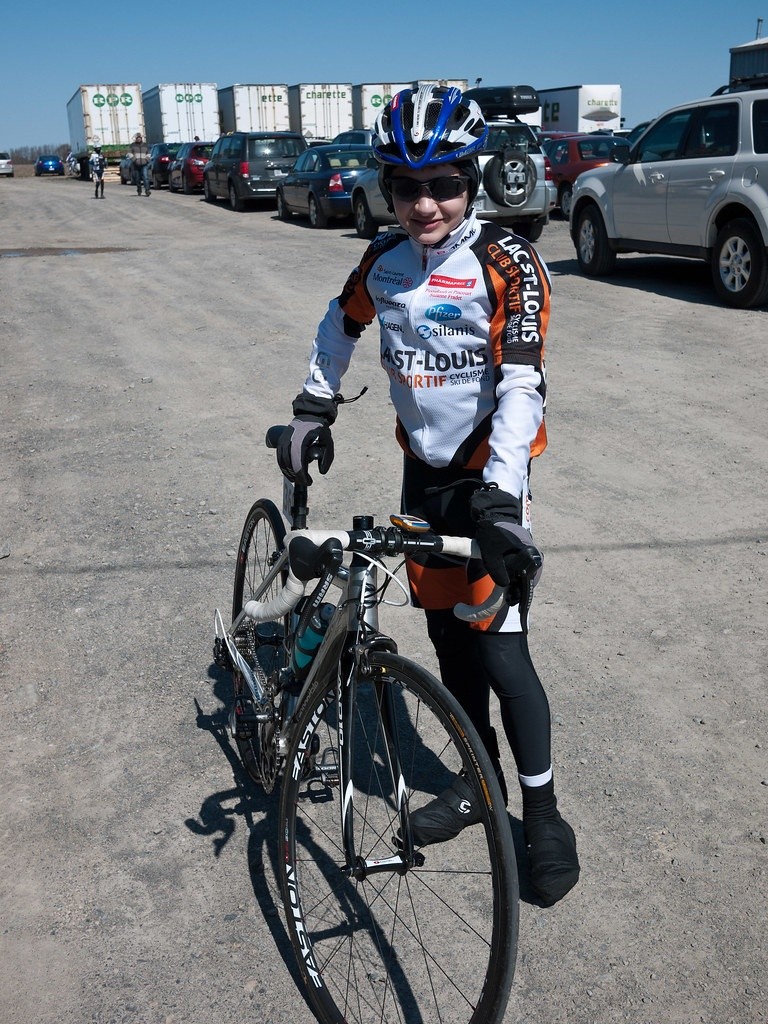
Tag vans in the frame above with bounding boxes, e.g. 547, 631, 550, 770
203, 128, 311, 212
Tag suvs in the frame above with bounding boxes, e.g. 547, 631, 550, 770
332, 128, 376, 150
167, 141, 218, 194
351, 86, 558, 242
568, 87, 768, 309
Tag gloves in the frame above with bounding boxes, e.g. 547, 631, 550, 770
277, 393, 338, 486
470, 488, 544, 606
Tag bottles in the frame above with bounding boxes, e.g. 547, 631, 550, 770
290, 603, 336, 674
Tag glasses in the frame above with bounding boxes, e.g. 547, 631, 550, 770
381, 175, 470, 202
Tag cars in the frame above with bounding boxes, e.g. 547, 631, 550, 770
283, 138, 333, 155
538, 117, 689, 164
146, 143, 181, 190
0, 153, 13, 177
276, 144, 373, 228
119, 154, 135, 185
34, 155, 64, 176
541, 135, 634, 218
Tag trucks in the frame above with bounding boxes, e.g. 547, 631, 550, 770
354, 79, 467, 133
289, 83, 354, 145
217, 84, 289, 146
66, 84, 146, 166
143, 83, 221, 152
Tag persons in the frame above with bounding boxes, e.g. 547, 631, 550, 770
89, 144, 108, 199
66, 153, 77, 175
128, 133, 151, 196
278, 84, 582, 907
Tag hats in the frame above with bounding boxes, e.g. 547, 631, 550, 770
135, 132, 142, 138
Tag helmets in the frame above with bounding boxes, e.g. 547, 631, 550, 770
370, 84, 489, 169
93, 143, 102, 148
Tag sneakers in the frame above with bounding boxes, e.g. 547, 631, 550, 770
408, 764, 508, 845
522, 811, 580, 902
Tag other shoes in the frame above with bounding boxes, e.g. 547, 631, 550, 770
101, 195, 105, 199
95, 190, 98, 197
146, 190, 151, 196
138, 192, 141, 196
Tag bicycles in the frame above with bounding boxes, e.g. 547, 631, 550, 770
213, 425, 520, 1024
